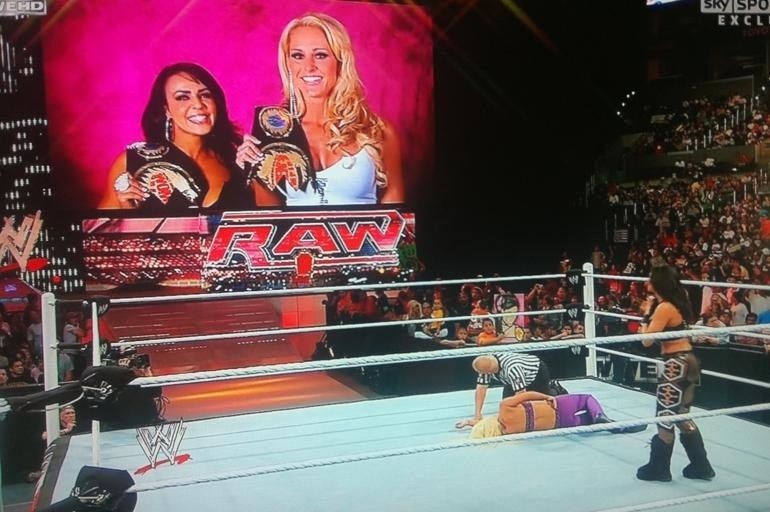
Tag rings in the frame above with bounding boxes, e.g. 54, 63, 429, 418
112, 173, 131, 193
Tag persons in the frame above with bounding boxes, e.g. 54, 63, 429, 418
0, 302, 165, 484
455, 351, 569, 429
471, 389, 649, 439
235, 11, 405, 207
634, 261, 716, 482
94, 60, 286, 211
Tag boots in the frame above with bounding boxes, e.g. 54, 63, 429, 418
678, 430, 715, 479
636, 434, 673, 482
594, 413, 648, 435
549, 379, 568, 395
